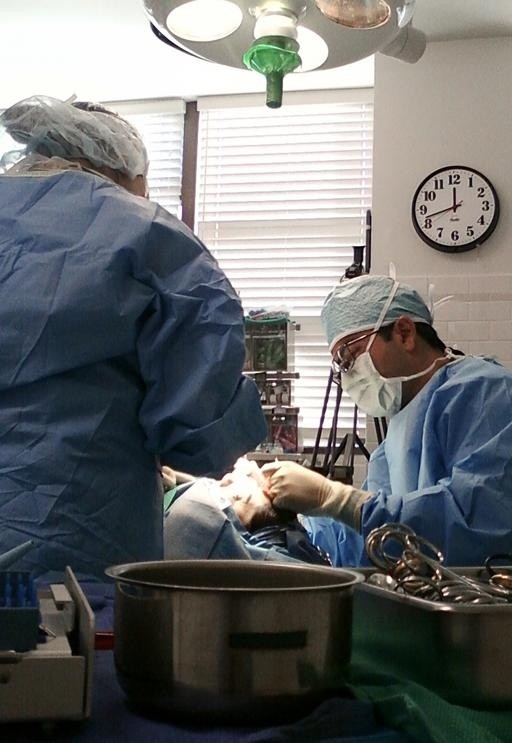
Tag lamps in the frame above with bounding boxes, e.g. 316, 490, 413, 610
142, 0, 426, 108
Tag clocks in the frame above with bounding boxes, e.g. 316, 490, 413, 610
411, 165, 499, 253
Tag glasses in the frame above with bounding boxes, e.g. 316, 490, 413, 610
331, 328, 387, 374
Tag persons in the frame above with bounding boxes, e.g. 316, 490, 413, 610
262, 274, 511, 567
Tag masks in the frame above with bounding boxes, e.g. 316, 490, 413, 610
340, 320, 423, 417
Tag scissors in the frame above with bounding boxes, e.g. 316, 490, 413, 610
366, 523, 512, 604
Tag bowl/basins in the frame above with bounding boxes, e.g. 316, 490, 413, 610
103, 557, 366, 729
